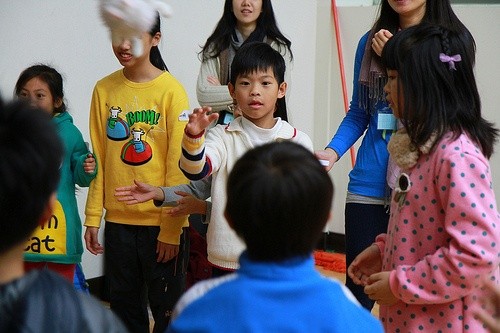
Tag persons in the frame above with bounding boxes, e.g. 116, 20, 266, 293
0, 99, 132, 332
167, 138, 386, 332
178, 41, 317, 283
12, 63, 99, 288
472, 280, 499, 332
311, 0, 478, 312
196, 0, 294, 129
82, 6, 191, 333
346, 24, 500, 333
